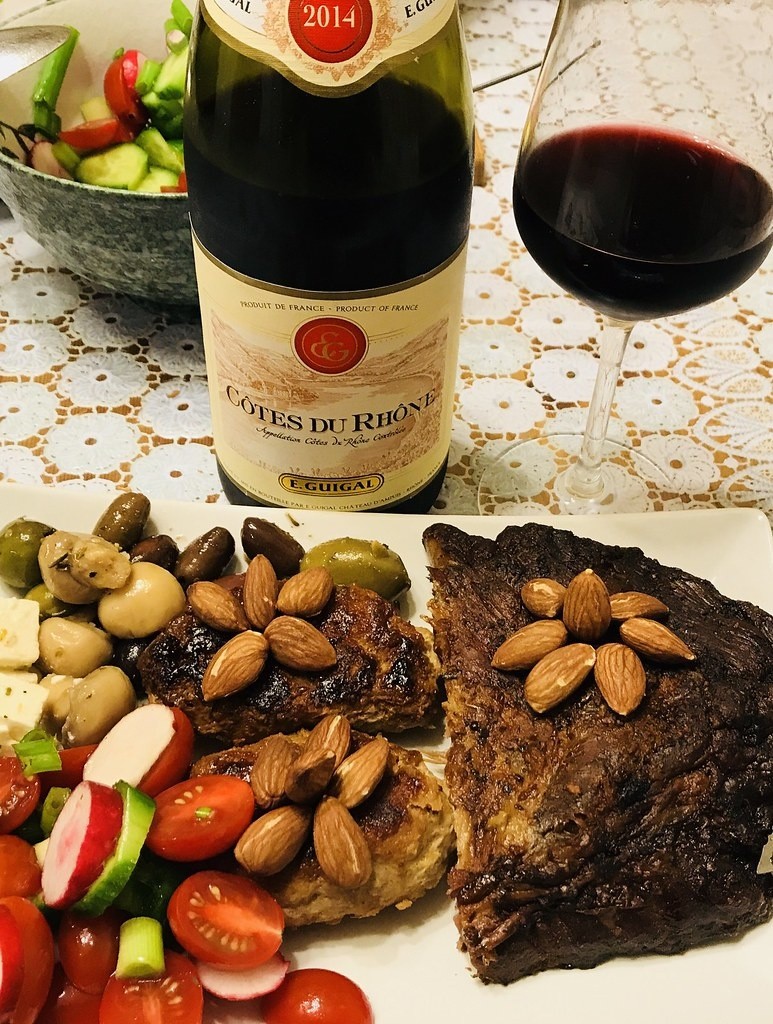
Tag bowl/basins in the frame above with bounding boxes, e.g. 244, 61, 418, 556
4, 5, 199, 304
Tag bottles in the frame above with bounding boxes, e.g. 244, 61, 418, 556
171, 0, 476, 514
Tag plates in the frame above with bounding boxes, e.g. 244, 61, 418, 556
4, 482, 771, 1024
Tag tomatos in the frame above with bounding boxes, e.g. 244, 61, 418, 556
0, 705, 373, 1024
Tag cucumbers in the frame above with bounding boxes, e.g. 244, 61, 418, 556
28, 0, 194, 194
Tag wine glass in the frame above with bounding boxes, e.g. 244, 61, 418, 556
513, 2, 772, 510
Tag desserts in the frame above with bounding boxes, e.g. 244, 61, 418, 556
135, 552, 446, 747
187, 716, 453, 927
418, 523, 773, 988
